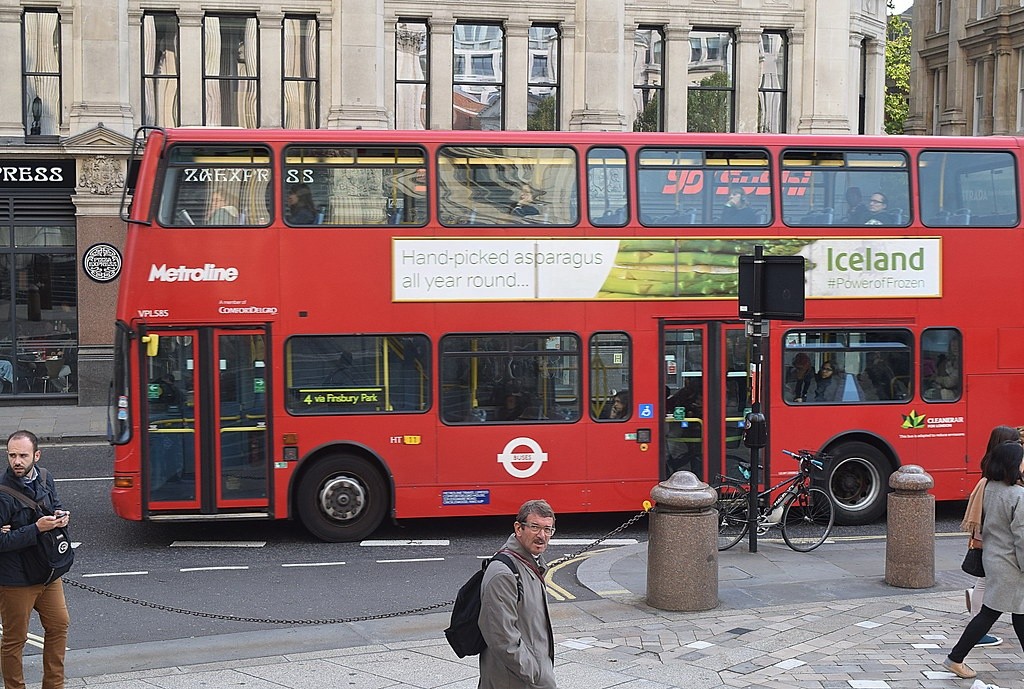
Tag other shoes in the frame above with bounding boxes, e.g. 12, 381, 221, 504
942, 657, 977, 678
973, 635, 1003, 648
965, 588, 976, 614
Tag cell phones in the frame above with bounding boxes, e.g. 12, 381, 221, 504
56, 514, 65, 519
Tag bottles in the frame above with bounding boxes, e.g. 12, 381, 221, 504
54, 319, 62, 331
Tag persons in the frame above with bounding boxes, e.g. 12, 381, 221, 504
0, 430, 70, 689
663, 379, 704, 480
784, 336, 961, 403
282, 184, 318, 226
55, 334, 76, 393
478, 500, 557, 689
942, 426, 1024, 677
206, 190, 241, 226
719, 187, 757, 225
326, 349, 525, 422
840, 186, 894, 225
504, 183, 539, 224
608, 392, 629, 420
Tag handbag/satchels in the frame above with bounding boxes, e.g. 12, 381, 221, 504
961, 538, 986, 577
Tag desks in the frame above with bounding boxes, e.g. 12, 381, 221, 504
18, 355, 57, 391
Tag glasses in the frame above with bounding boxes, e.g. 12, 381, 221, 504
820, 367, 833, 372
518, 522, 557, 537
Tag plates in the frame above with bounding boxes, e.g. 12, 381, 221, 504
47, 358, 59, 360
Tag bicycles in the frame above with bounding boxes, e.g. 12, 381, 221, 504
710, 449, 838, 554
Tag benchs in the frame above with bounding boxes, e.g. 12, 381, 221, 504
833, 373, 865, 403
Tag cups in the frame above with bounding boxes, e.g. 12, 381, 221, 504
42, 351, 46, 360
62, 323, 67, 331
47, 356, 58, 359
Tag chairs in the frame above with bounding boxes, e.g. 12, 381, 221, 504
1, 348, 77, 394
229, 205, 974, 228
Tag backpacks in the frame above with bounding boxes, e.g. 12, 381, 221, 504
0, 470, 75, 586
444, 554, 518, 660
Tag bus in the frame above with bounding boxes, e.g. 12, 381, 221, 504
107, 122, 1022, 543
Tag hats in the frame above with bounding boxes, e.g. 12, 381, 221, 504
791, 353, 811, 364
